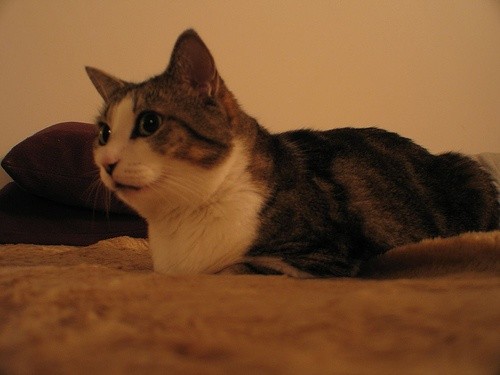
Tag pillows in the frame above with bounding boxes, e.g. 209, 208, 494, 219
1, 122, 142, 215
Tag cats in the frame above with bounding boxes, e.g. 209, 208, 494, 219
85, 28, 499, 275
